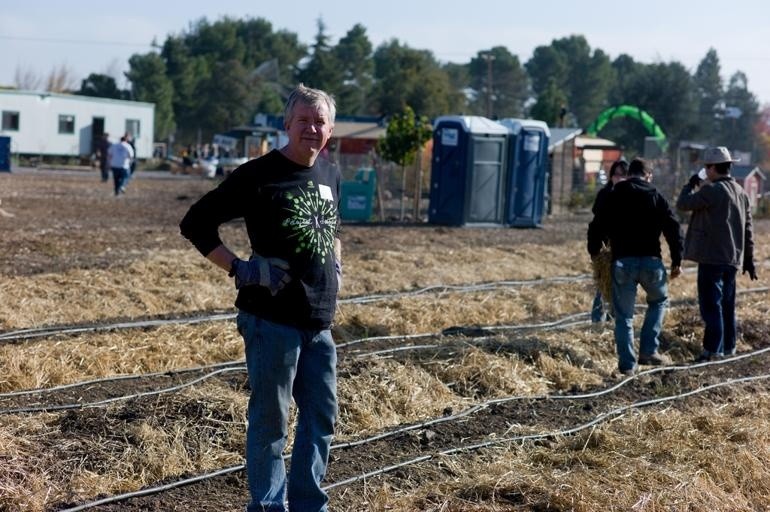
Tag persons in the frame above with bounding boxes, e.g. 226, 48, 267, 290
98, 132, 137, 195
676, 144, 758, 357
184, 140, 220, 178
587, 162, 629, 320
600, 158, 685, 374
179, 83, 344, 511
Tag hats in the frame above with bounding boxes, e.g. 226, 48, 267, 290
698, 147, 742, 164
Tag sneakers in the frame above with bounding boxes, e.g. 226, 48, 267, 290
621, 364, 638, 376
639, 352, 669, 366
696, 350, 725, 362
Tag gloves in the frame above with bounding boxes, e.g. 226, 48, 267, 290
743, 264, 759, 280
233, 258, 288, 290
334, 260, 342, 289
689, 173, 701, 188
